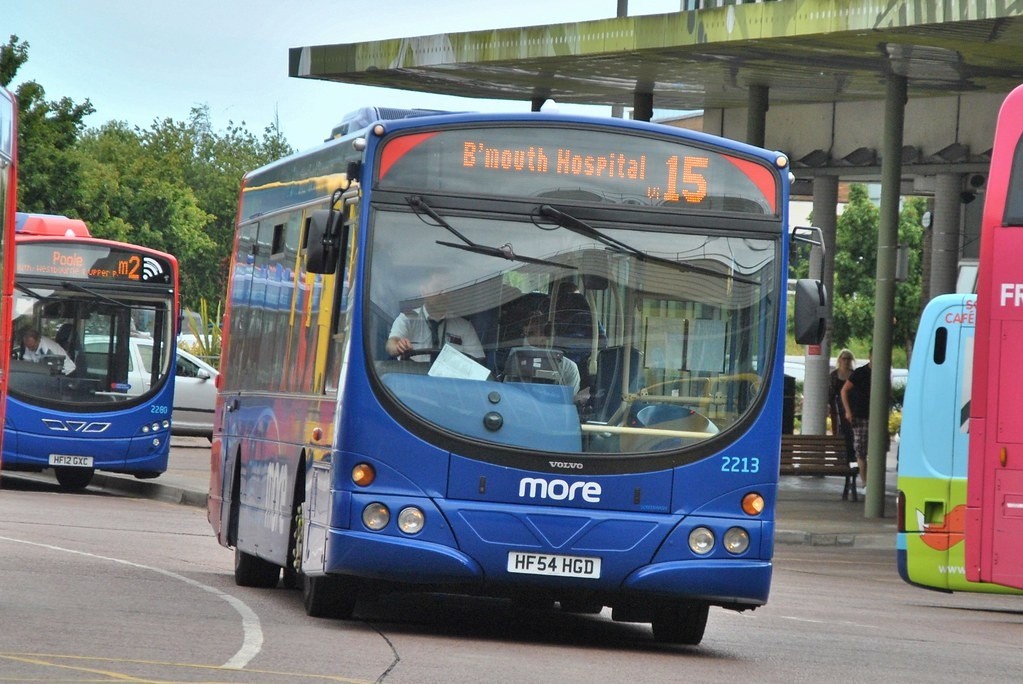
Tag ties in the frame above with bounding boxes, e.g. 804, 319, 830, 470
426, 318, 445, 363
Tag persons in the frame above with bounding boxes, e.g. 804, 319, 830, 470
841, 347, 891, 495
502, 315, 581, 398
382, 271, 486, 363
827, 349, 857, 467
557, 282, 580, 294
21, 329, 78, 379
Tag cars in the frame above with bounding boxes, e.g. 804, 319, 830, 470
71, 334, 220, 444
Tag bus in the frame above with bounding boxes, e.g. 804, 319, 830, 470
964, 82, 1023, 590
205, 112, 829, 647
0, 84, 19, 474
898, 294, 1023, 594
1, 212, 179, 490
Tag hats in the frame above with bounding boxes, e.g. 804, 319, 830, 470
521, 311, 552, 329
556, 272, 580, 291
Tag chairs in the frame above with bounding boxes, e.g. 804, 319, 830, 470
504, 286, 649, 418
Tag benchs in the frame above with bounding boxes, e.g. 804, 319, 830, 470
780, 435, 860, 501
